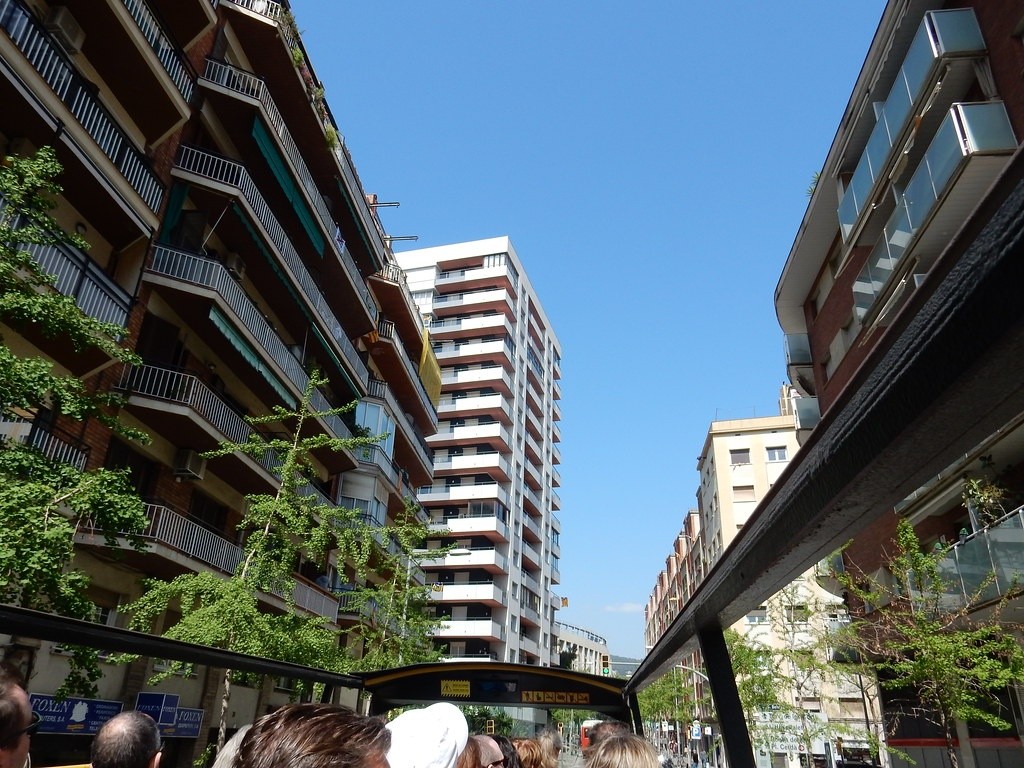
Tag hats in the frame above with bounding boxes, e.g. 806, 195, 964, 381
470, 735, 504, 767
382, 702, 468, 768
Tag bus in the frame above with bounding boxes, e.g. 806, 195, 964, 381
581, 720, 604, 759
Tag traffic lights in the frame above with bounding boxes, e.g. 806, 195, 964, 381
602, 655, 610, 675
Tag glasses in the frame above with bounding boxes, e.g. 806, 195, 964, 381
21, 711, 41, 735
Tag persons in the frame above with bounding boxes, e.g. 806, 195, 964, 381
0, 661, 663, 768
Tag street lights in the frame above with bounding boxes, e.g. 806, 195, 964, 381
398, 549, 473, 670
